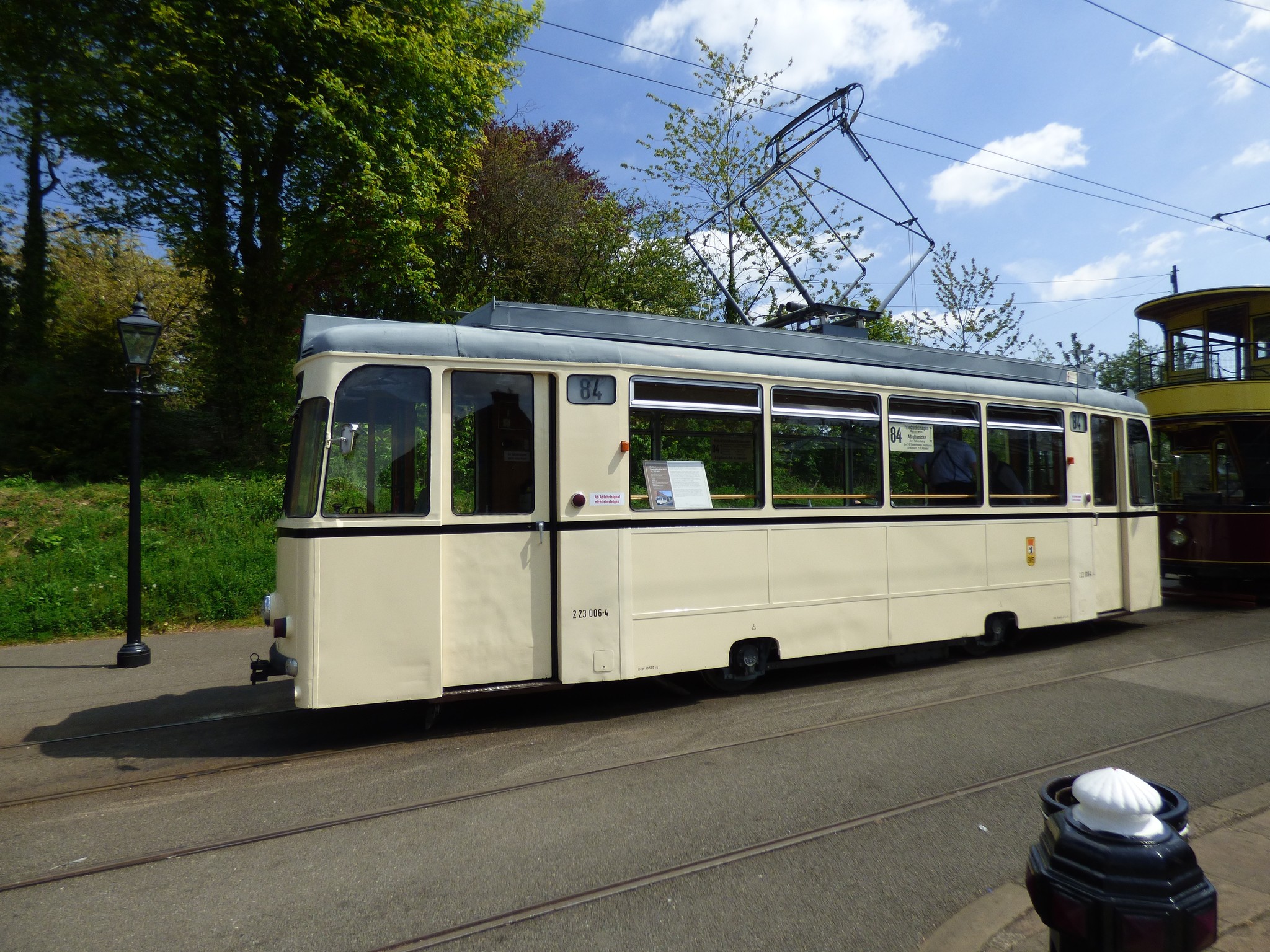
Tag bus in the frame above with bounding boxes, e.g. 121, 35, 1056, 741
1132, 202, 1270, 604
251, 83, 1167, 712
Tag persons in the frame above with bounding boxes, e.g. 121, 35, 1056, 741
987, 453, 1026, 505
912, 425, 978, 505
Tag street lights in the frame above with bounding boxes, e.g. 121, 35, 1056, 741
101, 291, 163, 669
